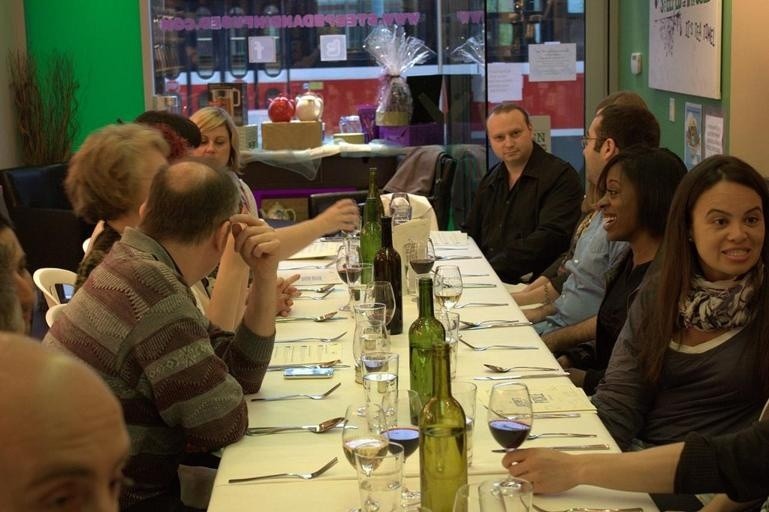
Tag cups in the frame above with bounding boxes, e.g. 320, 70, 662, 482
452, 478, 533, 511
450, 381, 477, 467
435, 311, 458, 380
354, 444, 404, 512
211, 88, 241, 119
360, 352, 398, 434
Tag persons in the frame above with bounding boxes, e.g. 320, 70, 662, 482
2, 106, 361, 511
502, 92, 768, 512
458, 103, 581, 292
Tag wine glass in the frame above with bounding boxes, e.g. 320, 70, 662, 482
381, 390, 420, 505
338, 192, 465, 365
488, 382, 531, 499
342, 403, 388, 512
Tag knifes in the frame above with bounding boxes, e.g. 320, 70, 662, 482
248, 424, 359, 431
271, 364, 351, 369
491, 444, 612, 452
278, 317, 348, 320
475, 371, 571, 381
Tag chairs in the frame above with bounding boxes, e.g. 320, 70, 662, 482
307, 145, 460, 231
0, 161, 96, 329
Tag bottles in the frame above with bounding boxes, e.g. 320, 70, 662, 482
359, 167, 390, 284
165, 97, 179, 115
419, 341, 468, 512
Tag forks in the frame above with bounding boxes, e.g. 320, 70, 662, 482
275, 331, 347, 342
296, 288, 335, 300
483, 405, 583, 419
533, 504, 644, 512
228, 457, 339, 483
286, 260, 337, 270
251, 382, 342, 400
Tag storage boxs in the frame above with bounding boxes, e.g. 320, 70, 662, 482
236, 125, 258, 152
261, 119, 322, 152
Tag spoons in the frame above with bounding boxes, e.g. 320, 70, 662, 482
527, 432, 599, 440
483, 362, 559, 373
298, 283, 335, 293
276, 311, 338, 322
244, 416, 343, 434
267, 360, 338, 371
